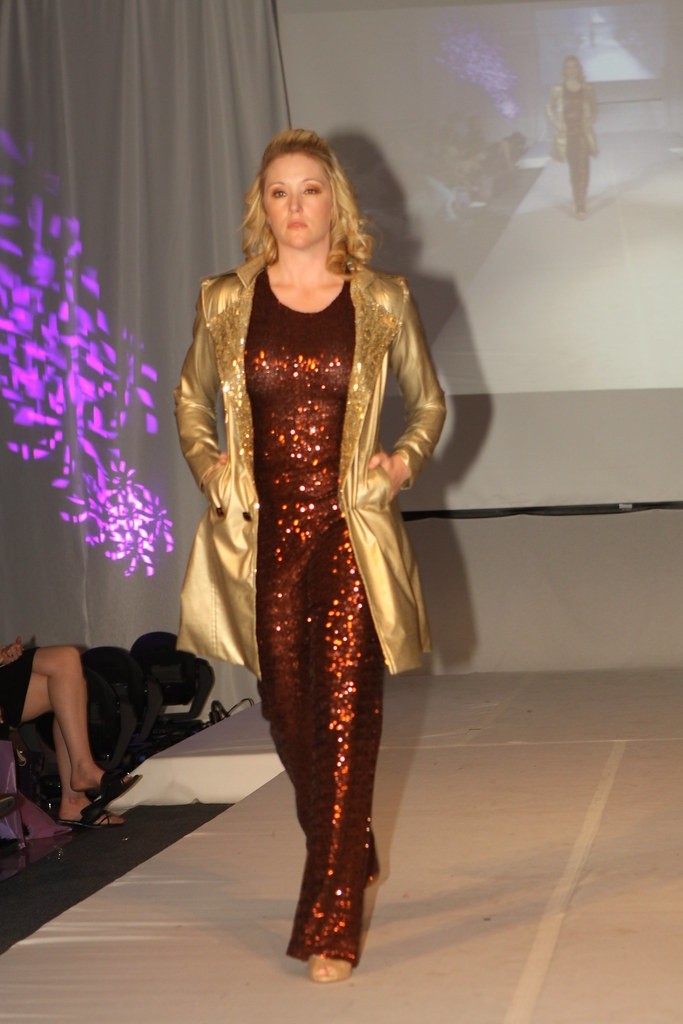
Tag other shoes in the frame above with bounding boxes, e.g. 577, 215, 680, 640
307, 955, 351, 981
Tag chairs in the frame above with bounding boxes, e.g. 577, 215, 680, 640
80, 632, 215, 768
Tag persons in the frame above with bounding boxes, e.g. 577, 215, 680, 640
175, 126, 445, 984
0, 634, 140, 829
544, 55, 600, 220
432, 117, 533, 215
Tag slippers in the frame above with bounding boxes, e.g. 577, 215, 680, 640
56, 808, 128, 829
80, 772, 141, 821
